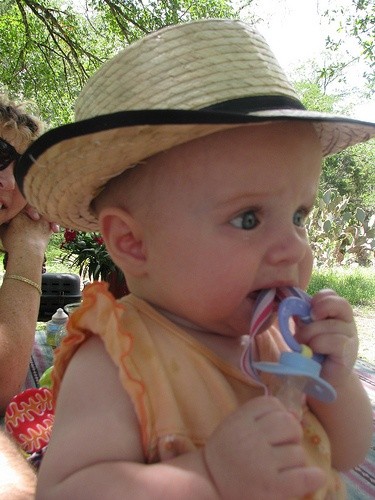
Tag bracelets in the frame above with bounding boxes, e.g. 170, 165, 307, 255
4, 273, 42, 295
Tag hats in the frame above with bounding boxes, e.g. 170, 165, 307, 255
13, 20, 375, 231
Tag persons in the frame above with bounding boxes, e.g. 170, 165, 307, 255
0, 94, 62, 404
11, 19, 375, 500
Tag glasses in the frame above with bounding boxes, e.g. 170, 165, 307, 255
0, 138, 22, 171
